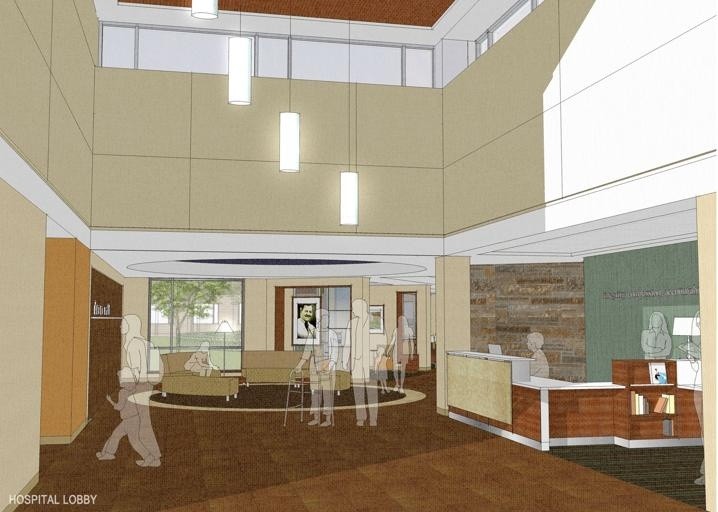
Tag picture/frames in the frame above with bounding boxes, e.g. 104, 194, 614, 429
291, 295, 323, 347
369, 304, 385, 334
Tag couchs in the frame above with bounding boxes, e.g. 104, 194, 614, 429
160, 352, 221, 378
241, 350, 321, 388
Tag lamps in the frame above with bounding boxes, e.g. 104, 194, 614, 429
191, 0, 361, 227
672, 316, 701, 360
215, 320, 234, 376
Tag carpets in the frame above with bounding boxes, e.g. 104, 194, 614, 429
148, 385, 408, 409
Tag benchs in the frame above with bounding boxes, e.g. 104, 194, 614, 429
161, 376, 240, 401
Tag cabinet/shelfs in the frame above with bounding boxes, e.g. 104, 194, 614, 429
611, 358, 677, 441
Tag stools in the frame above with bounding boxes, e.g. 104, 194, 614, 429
312, 370, 352, 395
